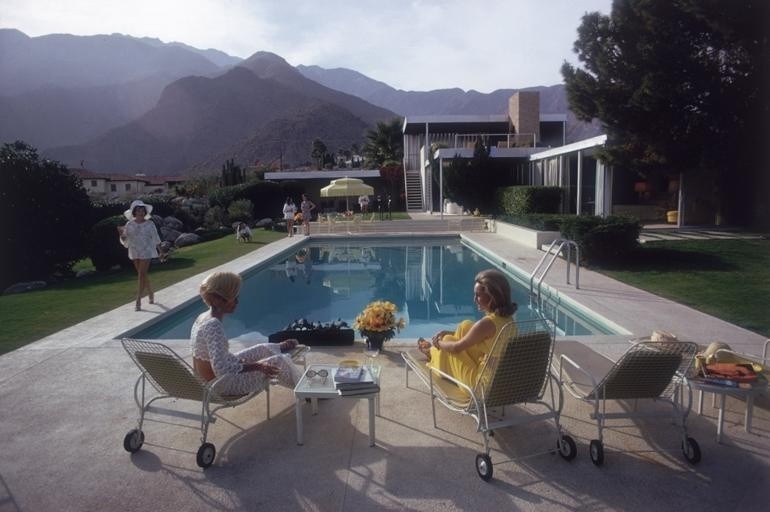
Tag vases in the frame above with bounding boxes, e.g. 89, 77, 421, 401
360, 327, 397, 353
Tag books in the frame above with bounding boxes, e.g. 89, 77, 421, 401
701, 361, 757, 390
332, 365, 380, 398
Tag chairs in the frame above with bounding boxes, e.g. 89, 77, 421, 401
318, 243, 377, 264
628, 327, 770, 419
273, 205, 381, 235
545, 331, 709, 468
397, 314, 578, 483
114, 335, 311, 468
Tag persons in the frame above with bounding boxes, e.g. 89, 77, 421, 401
188, 271, 326, 402
361, 248, 370, 267
286, 255, 297, 282
302, 248, 313, 285
300, 194, 316, 236
283, 196, 297, 237
419, 269, 517, 403
118, 200, 166, 310
359, 194, 369, 216
236, 222, 252, 243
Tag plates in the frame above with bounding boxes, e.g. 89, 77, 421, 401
338, 359, 360, 365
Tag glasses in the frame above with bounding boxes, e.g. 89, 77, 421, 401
306, 368, 328, 385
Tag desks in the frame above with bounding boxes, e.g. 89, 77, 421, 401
291, 360, 388, 451
669, 357, 770, 445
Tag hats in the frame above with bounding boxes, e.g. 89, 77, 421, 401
124, 200, 153, 220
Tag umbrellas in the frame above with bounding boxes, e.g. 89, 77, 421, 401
320, 176, 374, 211
322, 258, 369, 296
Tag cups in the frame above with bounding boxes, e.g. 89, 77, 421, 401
695, 355, 706, 367
269, 361, 281, 386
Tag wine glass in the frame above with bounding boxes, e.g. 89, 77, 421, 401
364, 346, 379, 376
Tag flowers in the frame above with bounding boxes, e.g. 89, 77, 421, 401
348, 300, 408, 337
